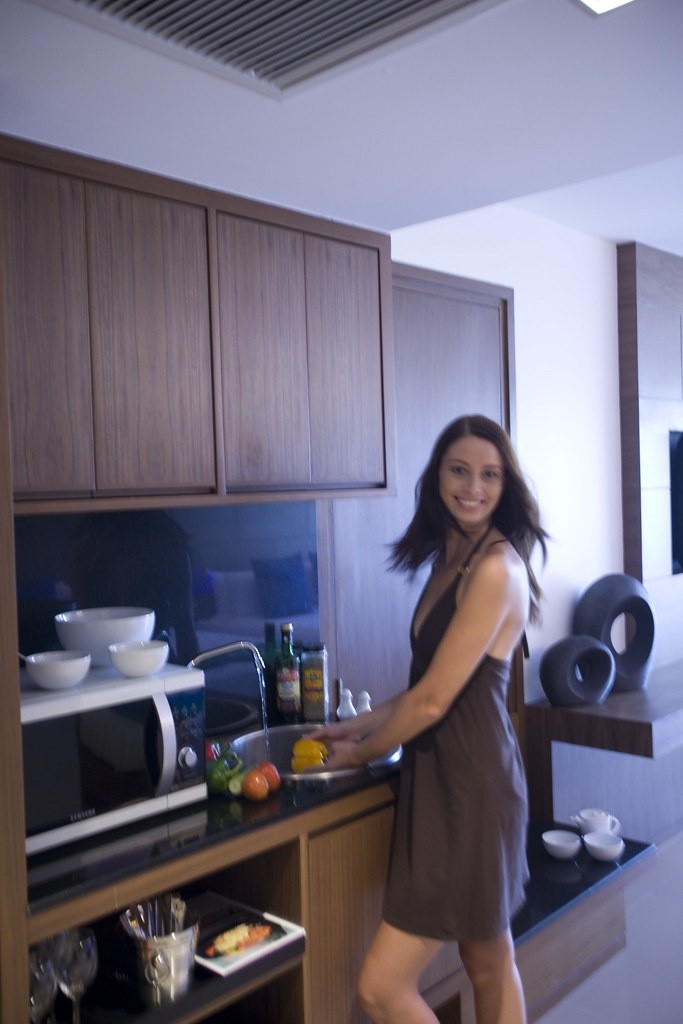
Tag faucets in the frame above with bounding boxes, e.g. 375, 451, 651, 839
186, 641, 267, 674
150, 629, 169, 641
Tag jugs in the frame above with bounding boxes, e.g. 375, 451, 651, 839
569, 808, 621, 836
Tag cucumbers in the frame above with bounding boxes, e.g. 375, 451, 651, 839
228, 767, 251, 795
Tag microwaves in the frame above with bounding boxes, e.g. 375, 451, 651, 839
19, 666, 209, 856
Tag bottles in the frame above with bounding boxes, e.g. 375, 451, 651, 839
357, 690, 372, 720
273, 621, 303, 727
299, 641, 329, 725
340, 687, 356, 722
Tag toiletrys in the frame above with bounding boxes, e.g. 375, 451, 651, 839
336, 687, 357, 722
355, 690, 372, 714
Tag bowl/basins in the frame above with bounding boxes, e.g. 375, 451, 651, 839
542, 830, 581, 860
108, 639, 169, 680
54, 605, 156, 667
25, 650, 92, 691
583, 831, 624, 864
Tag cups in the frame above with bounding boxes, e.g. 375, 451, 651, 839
139, 921, 200, 1010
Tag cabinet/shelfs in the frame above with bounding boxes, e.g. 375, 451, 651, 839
215, 172, 390, 493
213, 799, 469, 1024
7, 135, 216, 500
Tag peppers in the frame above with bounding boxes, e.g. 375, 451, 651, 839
204, 739, 243, 794
290, 737, 328, 773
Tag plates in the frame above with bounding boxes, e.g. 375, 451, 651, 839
185, 887, 305, 978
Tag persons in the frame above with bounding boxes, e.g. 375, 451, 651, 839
299, 414, 558, 1024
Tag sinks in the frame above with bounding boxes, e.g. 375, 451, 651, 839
203, 695, 258, 735
225, 722, 402, 779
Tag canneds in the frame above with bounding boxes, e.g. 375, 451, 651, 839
300, 642, 327, 722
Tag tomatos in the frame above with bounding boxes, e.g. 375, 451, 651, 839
241, 760, 280, 801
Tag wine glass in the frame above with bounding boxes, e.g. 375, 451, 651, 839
26, 926, 98, 1024
